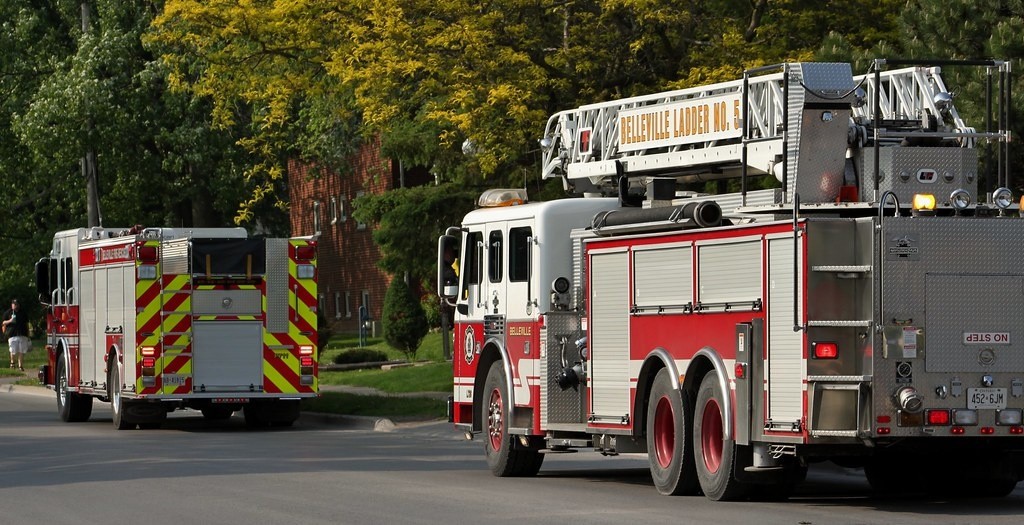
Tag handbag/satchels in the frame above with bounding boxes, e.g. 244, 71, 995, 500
2, 324, 7, 333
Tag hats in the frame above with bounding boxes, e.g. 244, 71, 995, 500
11, 298, 21, 304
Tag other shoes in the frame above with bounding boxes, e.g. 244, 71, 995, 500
9, 362, 16, 370
17, 364, 25, 371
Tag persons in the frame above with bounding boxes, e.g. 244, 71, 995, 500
443, 242, 468, 299
2, 299, 30, 371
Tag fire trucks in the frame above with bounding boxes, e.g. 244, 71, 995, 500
34, 226, 319, 432
437, 58, 1024, 501
